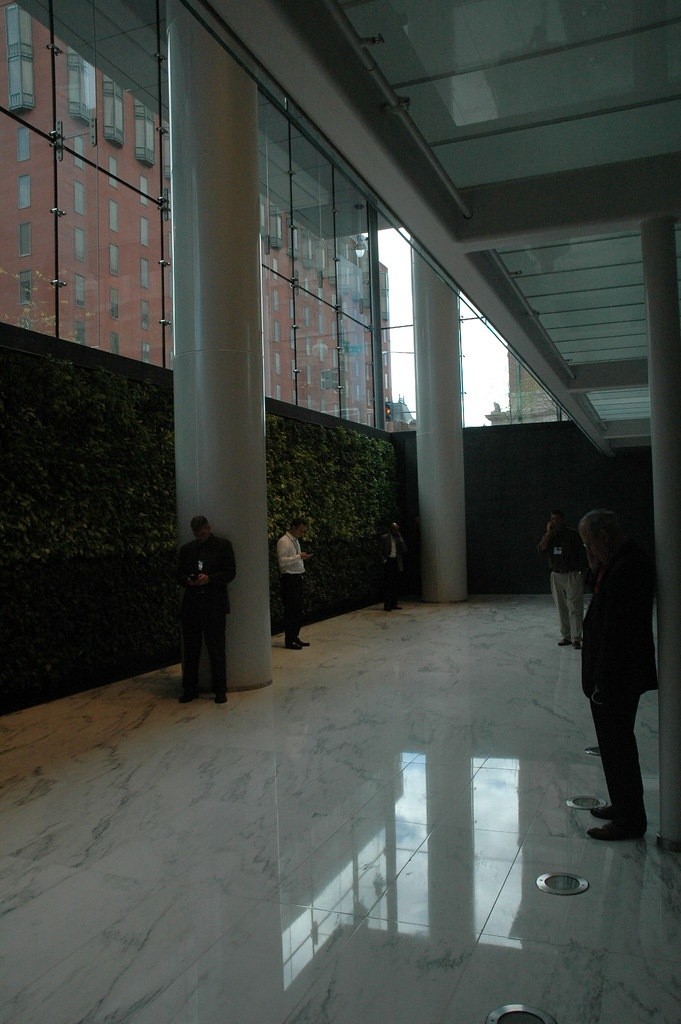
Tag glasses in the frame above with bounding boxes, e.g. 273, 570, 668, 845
583, 533, 604, 551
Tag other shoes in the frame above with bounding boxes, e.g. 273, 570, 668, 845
558, 638, 572, 646
214, 692, 227, 703
574, 641, 581, 649
178, 686, 197, 703
384, 606, 402, 611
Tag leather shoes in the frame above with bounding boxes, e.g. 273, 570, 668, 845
285, 637, 310, 650
586, 820, 646, 840
591, 803, 617, 820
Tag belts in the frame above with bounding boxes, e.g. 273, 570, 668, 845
553, 568, 578, 573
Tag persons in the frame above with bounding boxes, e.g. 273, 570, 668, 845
578, 509, 657, 840
378, 522, 407, 611
178, 516, 236, 703
277, 517, 313, 649
537, 511, 588, 648
